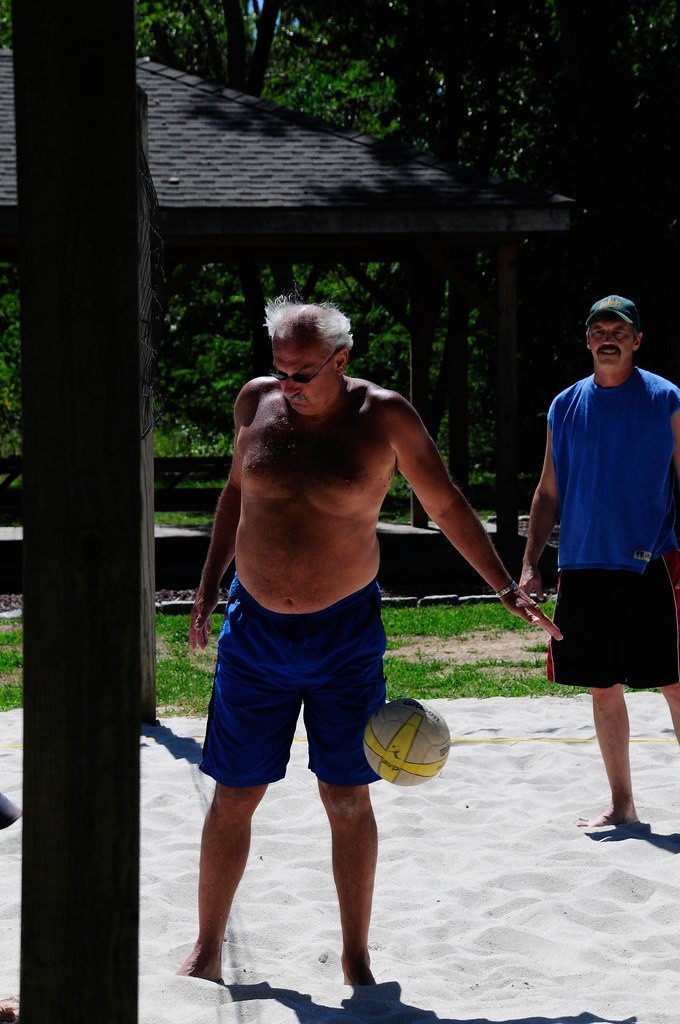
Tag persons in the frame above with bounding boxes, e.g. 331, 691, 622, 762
177, 300, 563, 986
520, 294, 680, 827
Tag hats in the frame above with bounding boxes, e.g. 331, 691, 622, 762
585, 295, 640, 330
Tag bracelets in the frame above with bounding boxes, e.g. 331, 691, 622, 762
496, 581, 516, 598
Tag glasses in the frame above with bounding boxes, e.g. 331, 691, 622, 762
269, 347, 342, 384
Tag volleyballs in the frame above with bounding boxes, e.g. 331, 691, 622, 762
364, 700, 448, 792
